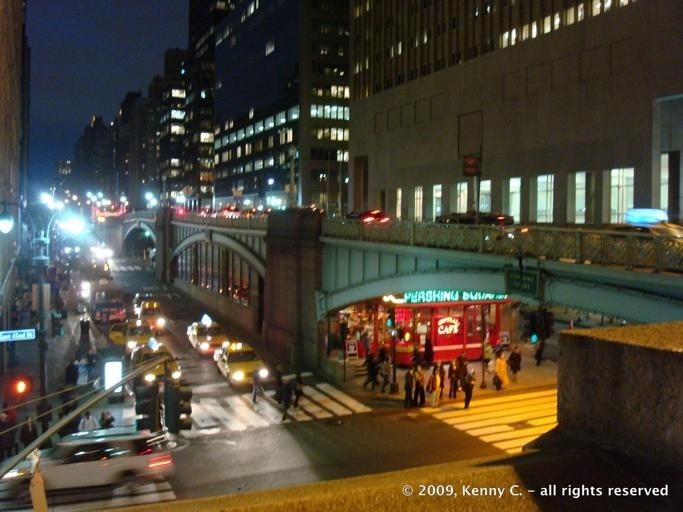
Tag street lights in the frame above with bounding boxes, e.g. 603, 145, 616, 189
266, 177, 274, 208
288, 143, 299, 209
70, 185, 189, 228
0, 185, 87, 450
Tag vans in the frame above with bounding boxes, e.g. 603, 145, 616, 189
0, 423, 177, 500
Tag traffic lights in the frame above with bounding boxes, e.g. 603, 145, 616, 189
14, 375, 32, 396
541, 309, 555, 342
523, 311, 538, 344
387, 307, 396, 327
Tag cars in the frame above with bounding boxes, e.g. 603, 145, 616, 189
593, 207, 682, 276
196, 203, 393, 229
57, 232, 273, 393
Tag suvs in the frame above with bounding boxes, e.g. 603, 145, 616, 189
435, 208, 529, 253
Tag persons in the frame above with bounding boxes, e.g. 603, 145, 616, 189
362, 330, 522, 411
534, 337, 544, 366
252, 364, 265, 403
272, 362, 285, 404
275, 375, 297, 411
0, 395, 116, 461
6, 248, 67, 368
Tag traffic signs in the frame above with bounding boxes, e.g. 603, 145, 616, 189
503, 265, 543, 299
